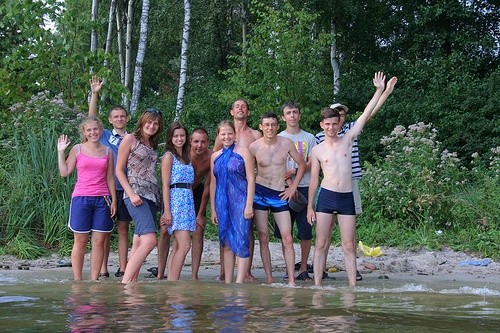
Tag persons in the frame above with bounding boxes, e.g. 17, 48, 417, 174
89, 74, 133, 276
315, 76, 398, 281
274, 101, 316, 282
161, 122, 197, 280
210, 120, 255, 283
213, 99, 263, 282
307, 70, 386, 287
56, 116, 116, 281
157, 127, 215, 279
115, 109, 163, 283
247, 112, 305, 286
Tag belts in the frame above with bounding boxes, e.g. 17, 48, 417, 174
169, 183, 192, 190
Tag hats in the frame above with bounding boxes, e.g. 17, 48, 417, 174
288, 191, 308, 212
329, 103, 349, 113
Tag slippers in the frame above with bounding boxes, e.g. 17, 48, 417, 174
283, 261, 362, 282
144, 273, 157, 278
147, 267, 158, 272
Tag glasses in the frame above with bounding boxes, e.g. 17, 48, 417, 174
143, 108, 163, 118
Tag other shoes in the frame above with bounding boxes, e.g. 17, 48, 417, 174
115, 268, 125, 277
98, 273, 110, 282
